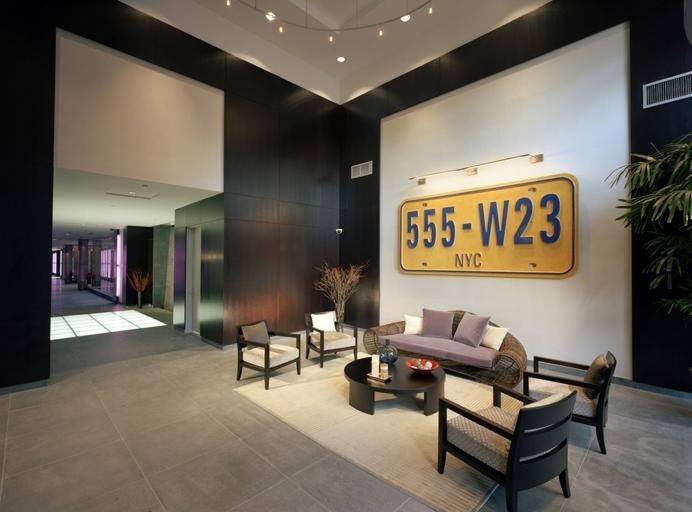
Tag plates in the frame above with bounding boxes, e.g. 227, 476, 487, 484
367, 371, 392, 382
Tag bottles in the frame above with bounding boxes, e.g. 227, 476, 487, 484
377, 339, 399, 364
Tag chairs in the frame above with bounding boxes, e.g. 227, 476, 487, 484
435, 350, 619, 512
304, 311, 359, 369
233, 318, 302, 390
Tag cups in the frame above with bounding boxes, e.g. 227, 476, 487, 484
380, 363, 388, 379
371, 354, 380, 377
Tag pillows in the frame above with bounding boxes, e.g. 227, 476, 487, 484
401, 305, 511, 351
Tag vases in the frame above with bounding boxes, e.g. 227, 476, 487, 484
137, 290, 143, 310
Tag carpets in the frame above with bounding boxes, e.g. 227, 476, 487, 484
234, 352, 521, 511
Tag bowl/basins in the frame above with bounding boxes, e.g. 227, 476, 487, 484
405, 358, 439, 374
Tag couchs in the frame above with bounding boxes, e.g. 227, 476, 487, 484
362, 310, 528, 390
